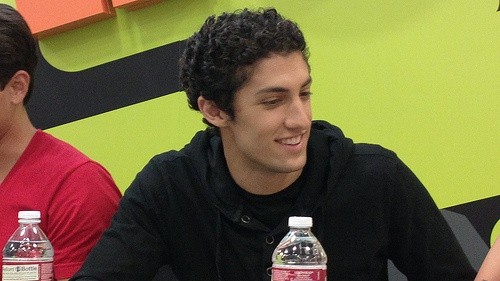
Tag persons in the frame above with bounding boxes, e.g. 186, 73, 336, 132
0, 4, 123, 281
69, 9, 480, 281
474, 219, 500, 281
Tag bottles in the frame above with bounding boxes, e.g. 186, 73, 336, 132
271, 217, 327, 281
1, 211, 54, 281
